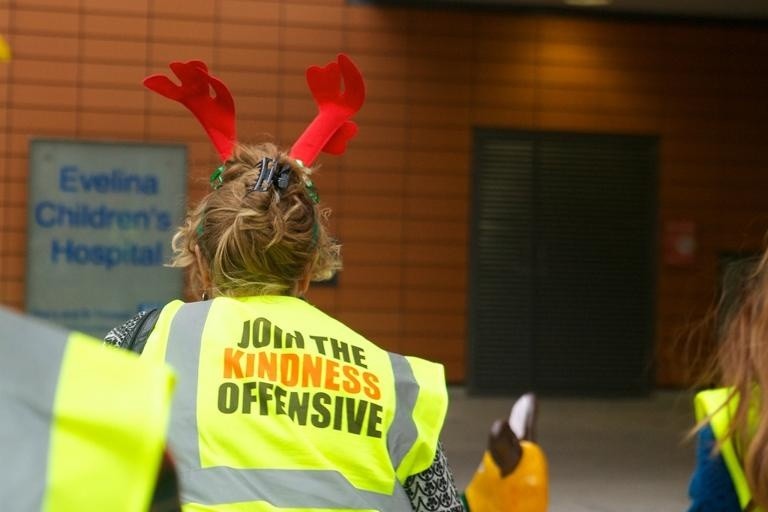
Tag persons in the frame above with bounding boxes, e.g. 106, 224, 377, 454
0, 300, 184, 512
685, 248, 768, 512
98, 142, 468, 512
